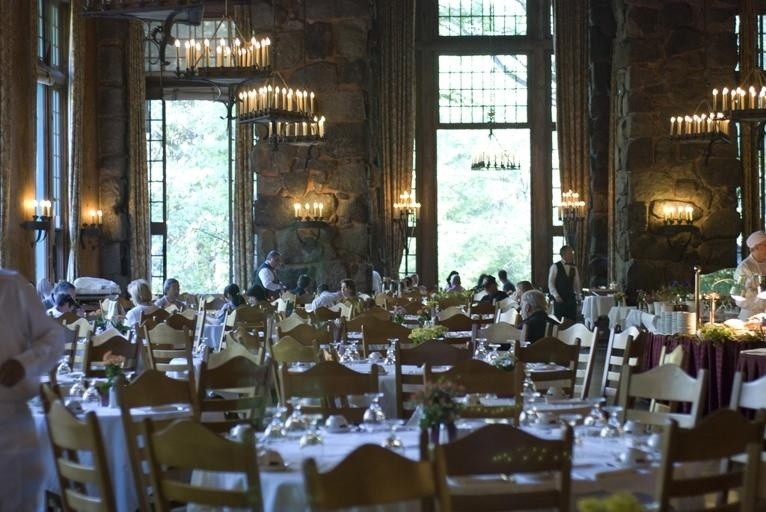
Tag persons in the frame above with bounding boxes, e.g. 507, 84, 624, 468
382, 271, 464, 293
42, 282, 81, 317
289, 274, 356, 309
123, 278, 187, 344
549, 246, 582, 321
472, 269, 561, 350
206, 282, 272, 324
0, 269, 64, 512
254, 250, 286, 300
731, 231, 766, 321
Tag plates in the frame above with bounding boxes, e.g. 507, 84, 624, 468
659, 310, 696, 336
367, 369, 388, 375
533, 365, 555, 370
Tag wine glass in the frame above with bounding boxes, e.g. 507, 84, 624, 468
263, 391, 628, 458
55, 354, 104, 411
195, 335, 210, 357
327, 335, 531, 368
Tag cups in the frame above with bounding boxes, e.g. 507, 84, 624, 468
226, 421, 285, 472
619, 418, 664, 467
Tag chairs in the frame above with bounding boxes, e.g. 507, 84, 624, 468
136, 412, 267, 512
609, 408, 766, 512
300, 425, 439, 511
119, 398, 204, 511
433, 422, 574, 510
28, 261, 766, 421
34, 379, 116, 512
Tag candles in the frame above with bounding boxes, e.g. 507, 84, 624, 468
387, 189, 423, 216
659, 203, 694, 226
165, 30, 328, 152
291, 199, 326, 225
666, 82, 765, 142
553, 189, 585, 222
30, 198, 105, 229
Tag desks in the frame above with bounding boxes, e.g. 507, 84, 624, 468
196, 401, 664, 505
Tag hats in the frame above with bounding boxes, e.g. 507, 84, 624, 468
745, 230, 766, 250
